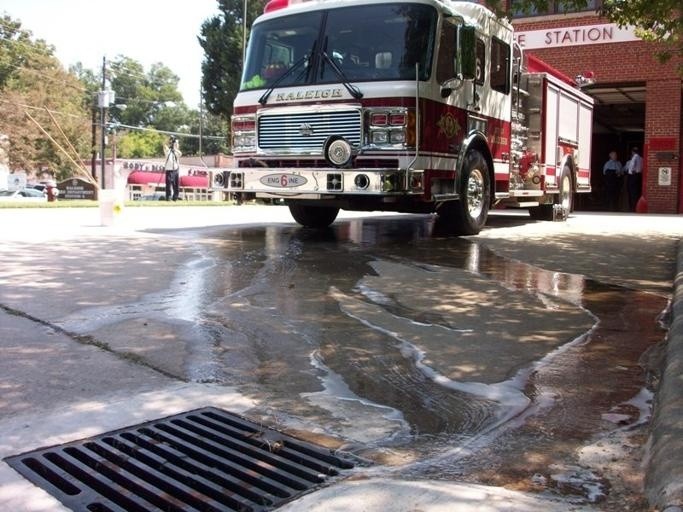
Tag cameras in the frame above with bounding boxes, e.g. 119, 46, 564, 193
171, 137, 176, 142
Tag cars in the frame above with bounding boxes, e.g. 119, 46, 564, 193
0, 187, 48, 200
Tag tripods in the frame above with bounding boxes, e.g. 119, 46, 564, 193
151, 147, 189, 201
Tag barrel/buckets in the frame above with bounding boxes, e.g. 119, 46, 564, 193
98, 189, 126, 224
210, 191, 222, 203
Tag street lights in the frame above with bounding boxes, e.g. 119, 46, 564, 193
102, 104, 127, 190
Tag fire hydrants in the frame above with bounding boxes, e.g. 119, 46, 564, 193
46, 183, 59, 201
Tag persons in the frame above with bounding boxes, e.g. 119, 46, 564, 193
160, 134, 182, 201
603, 147, 643, 212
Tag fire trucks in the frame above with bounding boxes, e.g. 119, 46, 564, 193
211, 1, 596, 234
